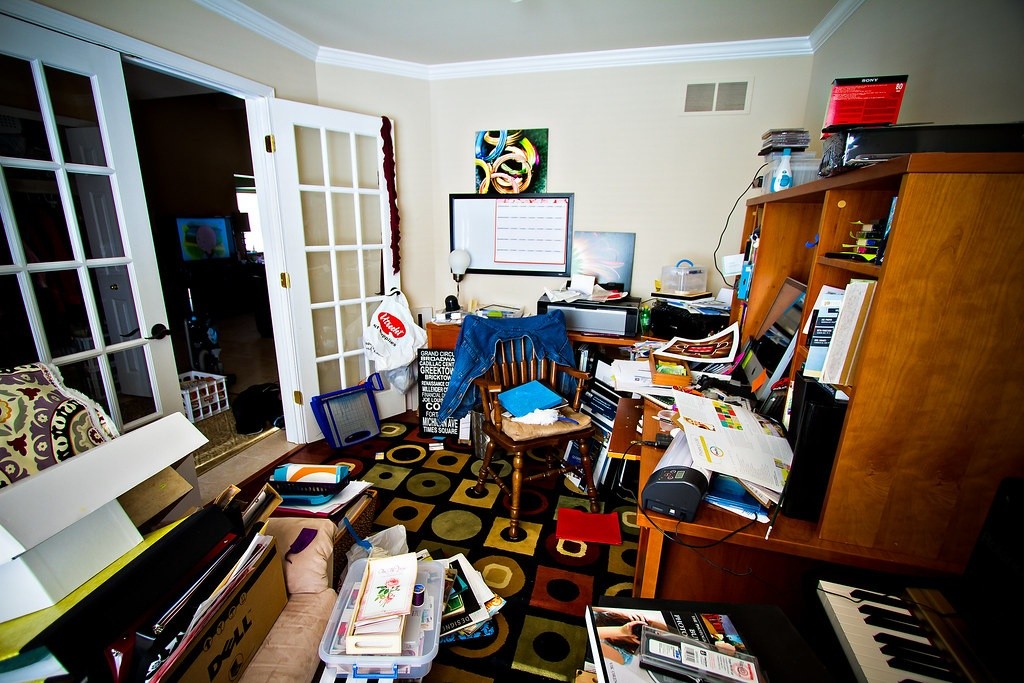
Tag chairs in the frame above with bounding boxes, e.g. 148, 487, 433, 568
472, 309, 601, 539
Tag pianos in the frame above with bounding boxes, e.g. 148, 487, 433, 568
784, 578, 966, 683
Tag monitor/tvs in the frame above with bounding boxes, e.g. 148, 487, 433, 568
176, 216, 235, 262
740, 276, 808, 401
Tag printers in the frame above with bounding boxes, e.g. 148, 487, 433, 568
640, 430, 713, 523
537, 280, 641, 337
650, 301, 730, 341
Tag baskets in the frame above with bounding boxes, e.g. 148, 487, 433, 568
265, 464, 352, 495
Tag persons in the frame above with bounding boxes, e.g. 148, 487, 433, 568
593, 605, 666, 666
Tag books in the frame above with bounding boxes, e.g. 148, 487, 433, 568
414, 551, 506, 638
666, 301, 732, 316
574, 344, 595, 385
733, 477, 783, 509
802, 278, 878, 387
584, 601, 757, 683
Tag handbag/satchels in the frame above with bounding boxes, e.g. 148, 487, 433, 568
363, 288, 428, 372
385, 365, 418, 395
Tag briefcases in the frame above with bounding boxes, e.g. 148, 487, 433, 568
659, 258, 708, 295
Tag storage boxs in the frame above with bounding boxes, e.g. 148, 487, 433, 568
178, 370, 230, 423
662, 267, 707, 295
0, 412, 446, 683
762, 158, 822, 194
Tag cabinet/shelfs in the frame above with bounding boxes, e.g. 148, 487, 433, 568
425, 152, 1024, 618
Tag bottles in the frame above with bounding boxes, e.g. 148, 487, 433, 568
639, 306, 651, 337
770, 148, 793, 192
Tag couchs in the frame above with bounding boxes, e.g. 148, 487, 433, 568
240, 518, 340, 683
